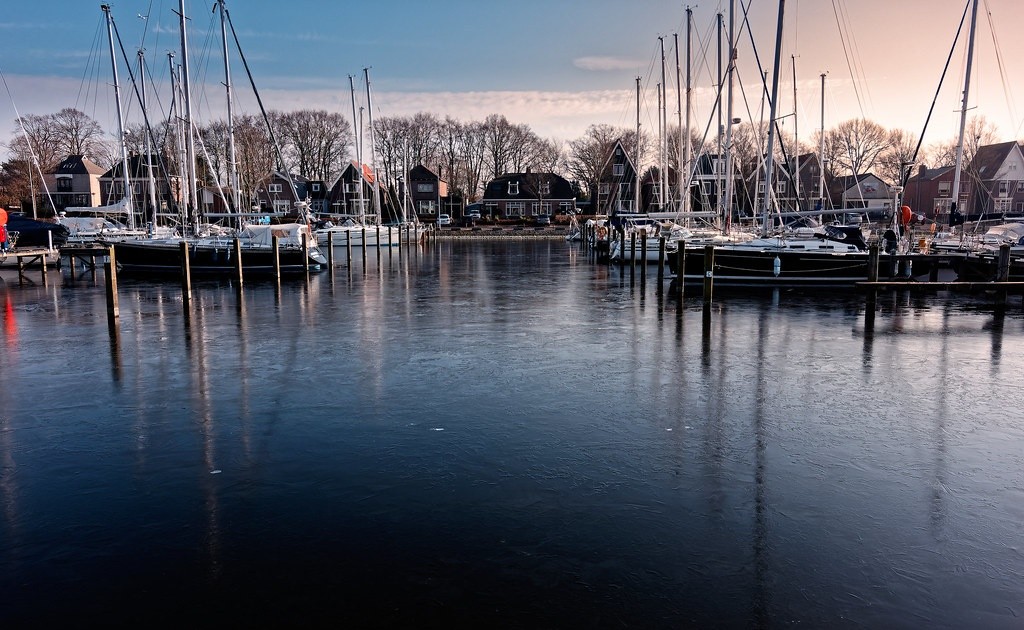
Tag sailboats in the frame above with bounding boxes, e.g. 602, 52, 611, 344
585, 1, 944, 310
308, 60, 426, 249
102, 0, 322, 270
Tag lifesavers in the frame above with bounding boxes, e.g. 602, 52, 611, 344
285, 246, 297, 258
599, 226, 607, 239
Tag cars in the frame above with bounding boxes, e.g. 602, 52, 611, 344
537, 212, 551, 226
469, 209, 481, 221
436, 214, 451, 227
